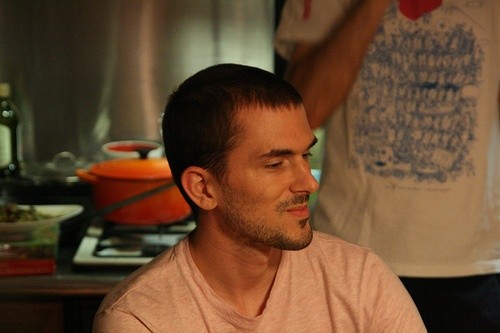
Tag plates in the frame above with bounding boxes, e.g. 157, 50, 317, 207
1, 204, 83, 241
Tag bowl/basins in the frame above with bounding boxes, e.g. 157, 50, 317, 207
102, 140, 163, 159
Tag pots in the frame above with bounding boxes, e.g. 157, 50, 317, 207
76, 148, 192, 226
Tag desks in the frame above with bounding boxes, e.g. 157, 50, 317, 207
1, 275, 134, 333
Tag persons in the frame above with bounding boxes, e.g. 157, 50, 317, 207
272, 0, 500, 333
92, 63, 429, 333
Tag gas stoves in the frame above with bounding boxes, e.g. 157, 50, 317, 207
73, 218, 196, 267
